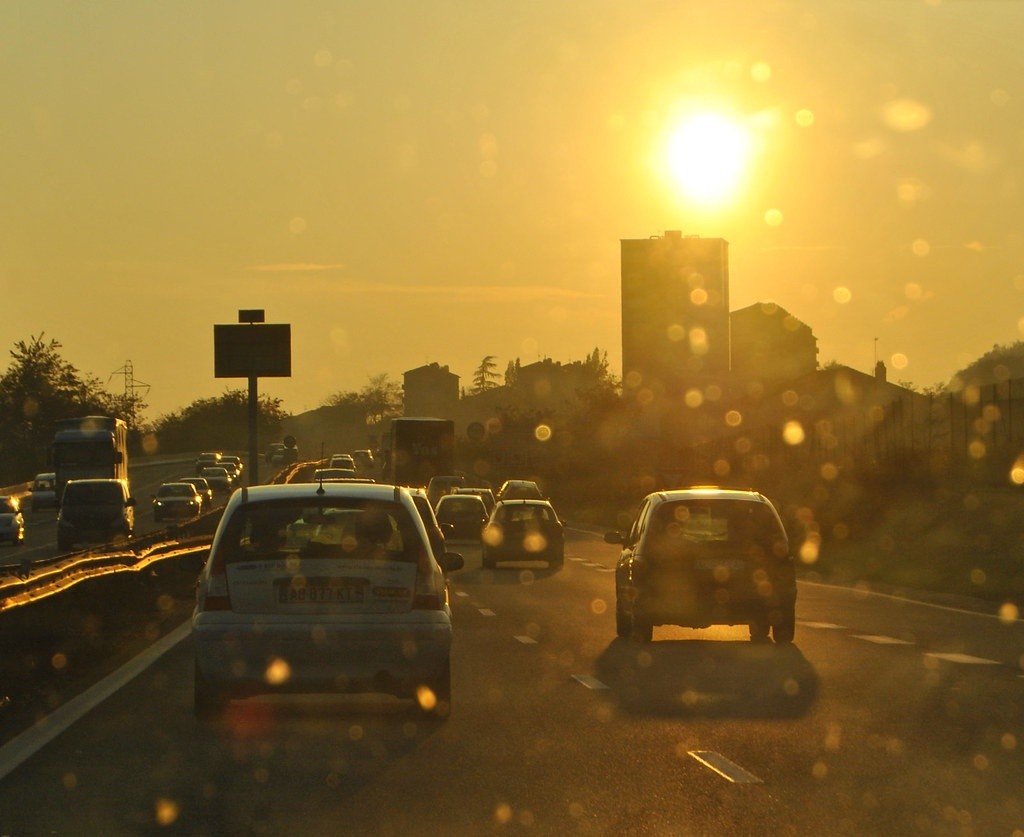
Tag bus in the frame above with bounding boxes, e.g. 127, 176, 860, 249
52, 415, 129, 477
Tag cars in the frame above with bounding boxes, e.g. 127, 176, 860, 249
433, 495, 489, 537
0, 495, 25, 544
190, 481, 465, 718
28, 472, 57, 512
331, 454, 351, 458
451, 487, 496, 515
314, 478, 376, 483
428, 476, 467, 506
195, 452, 243, 490
331, 458, 356, 469
57, 478, 137, 548
267, 442, 299, 466
482, 501, 566, 570
601, 486, 798, 643
405, 487, 455, 555
496, 480, 545, 501
180, 478, 212, 505
352, 449, 376, 466
314, 467, 354, 478
151, 481, 201, 518
